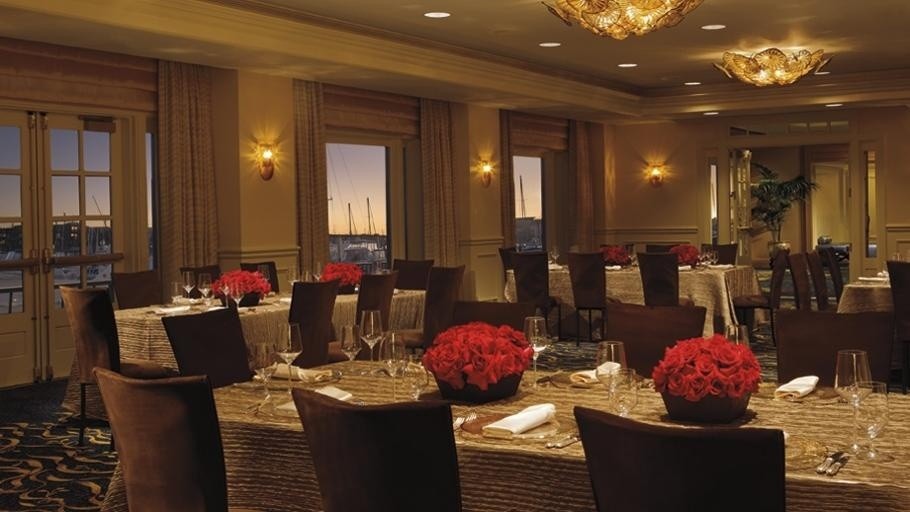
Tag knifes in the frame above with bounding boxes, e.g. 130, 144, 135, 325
232, 380, 288, 391
826, 454, 849, 476
545, 434, 580, 449
816, 451, 843, 474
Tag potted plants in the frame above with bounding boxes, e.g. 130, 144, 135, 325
735, 162, 823, 270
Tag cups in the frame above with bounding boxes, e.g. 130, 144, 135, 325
606, 366, 638, 417
401, 353, 430, 402
285, 261, 322, 289
595, 340, 629, 388
893, 253, 903, 261
169, 281, 183, 305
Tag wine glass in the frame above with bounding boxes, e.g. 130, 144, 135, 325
339, 323, 365, 376
693, 246, 720, 270
180, 267, 245, 313
630, 255, 637, 271
379, 332, 407, 404
832, 348, 875, 454
273, 321, 305, 395
523, 315, 549, 391
849, 381, 894, 464
547, 247, 560, 267
358, 309, 385, 378
250, 340, 279, 406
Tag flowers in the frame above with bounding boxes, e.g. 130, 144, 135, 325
600, 245, 629, 264
212, 268, 272, 301
422, 321, 535, 390
321, 260, 365, 286
667, 245, 699, 266
651, 333, 763, 406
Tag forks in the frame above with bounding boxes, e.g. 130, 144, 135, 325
453, 410, 478, 434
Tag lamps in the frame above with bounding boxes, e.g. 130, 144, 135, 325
648, 165, 663, 188
711, 48, 835, 87
539, 0, 705, 40
258, 142, 275, 181
480, 160, 492, 189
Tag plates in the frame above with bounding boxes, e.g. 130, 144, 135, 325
571, 381, 607, 389
155, 306, 190, 315
679, 266, 691, 271
856, 274, 887, 283
605, 264, 621, 271
548, 265, 563, 270
280, 298, 291, 304
708, 264, 732, 270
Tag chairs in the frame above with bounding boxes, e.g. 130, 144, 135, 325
886, 260, 908, 346
807, 251, 838, 311
389, 265, 467, 362
573, 404, 788, 511
566, 250, 623, 346
58, 285, 173, 454
161, 307, 253, 390
288, 277, 342, 370
392, 259, 434, 291
289, 387, 465, 509
239, 260, 281, 294
92, 366, 231, 510
730, 247, 791, 345
604, 297, 708, 382
509, 249, 563, 344
644, 245, 682, 254
452, 299, 538, 341
179, 264, 223, 300
787, 252, 813, 312
111, 271, 164, 309
498, 246, 516, 283
770, 306, 894, 396
821, 247, 847, 303
699, 244, 738, 266
636, 251, 694, 307
326, 270, 400, 365
